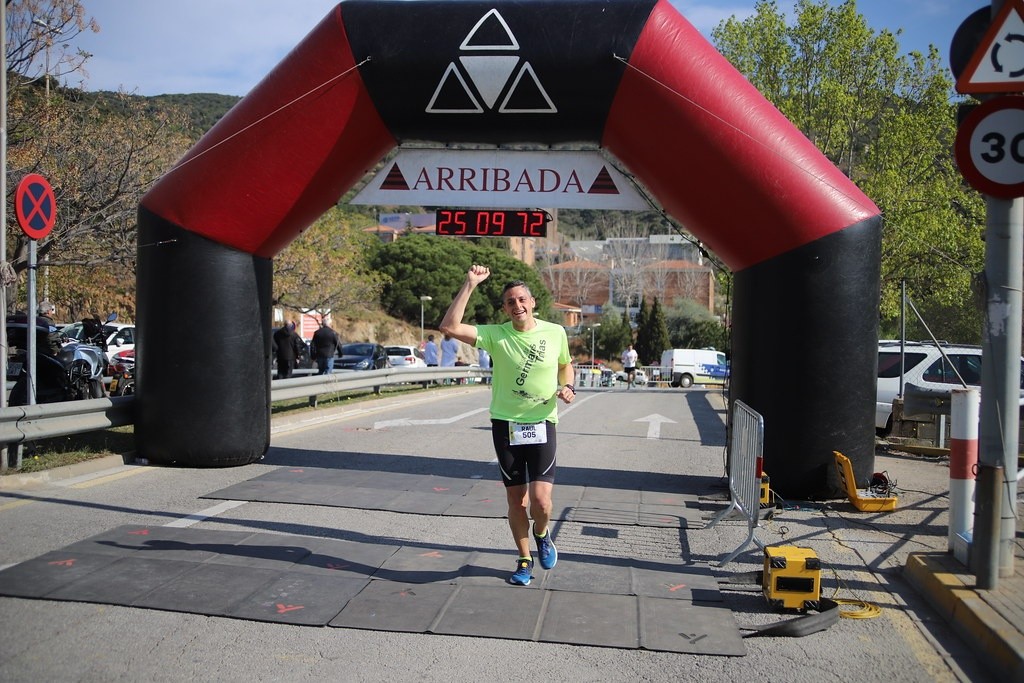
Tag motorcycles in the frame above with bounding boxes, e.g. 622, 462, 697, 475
9, 311, 119, 403
111, 350, 135, 396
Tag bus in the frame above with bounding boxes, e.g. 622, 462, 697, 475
272, 328, 319, 380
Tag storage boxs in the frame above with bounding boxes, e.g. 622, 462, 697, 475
832, 450, 898, 512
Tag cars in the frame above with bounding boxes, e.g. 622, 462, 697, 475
578, 361, 600, 369
616, 369, 648, 385
384, 346, 427, 367
454, 364, 482, 383
335, 343, 389, 370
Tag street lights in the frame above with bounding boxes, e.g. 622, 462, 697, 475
420, 295, 433, 341
592, 324, 602, 369
31, 16, 65, 315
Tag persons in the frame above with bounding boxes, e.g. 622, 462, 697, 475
39, 300, 63, 356
274, 320, 303, 379
425, 335, 438, 367
455, 357, 464, 384
439, 264, 576, 586
622, 344, 638, 390
310, 316, 344, 375
441, 335, 457, 367
478, 348, 490, 383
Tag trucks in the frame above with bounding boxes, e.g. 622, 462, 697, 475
576, 369, 617, 387
661, 346, 731, 388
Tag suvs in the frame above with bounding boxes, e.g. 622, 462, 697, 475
876, 339, 1024, 440
6, 320, 137, 353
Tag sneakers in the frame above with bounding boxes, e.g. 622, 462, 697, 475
532, 522, 557, 569
509, 555, 535, 586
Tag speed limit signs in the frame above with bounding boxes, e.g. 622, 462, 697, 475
954, 97, 1024, 199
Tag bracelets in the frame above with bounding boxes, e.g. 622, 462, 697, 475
564, 384, 577, 395
